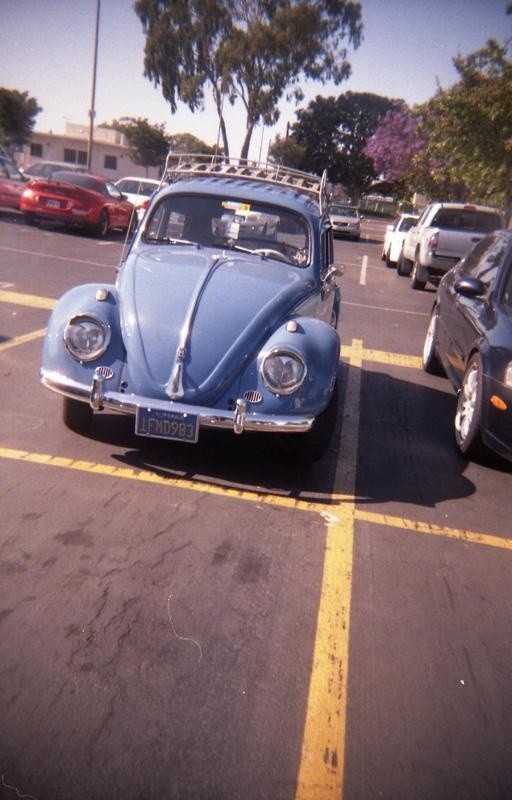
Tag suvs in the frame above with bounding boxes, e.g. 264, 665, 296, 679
395, 202, 509, 290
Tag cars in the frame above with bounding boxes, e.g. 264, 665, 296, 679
39, 149, 342, 445
421, 229, 512, 464
329, 204, 364, 240
382, 214, 421, 267
